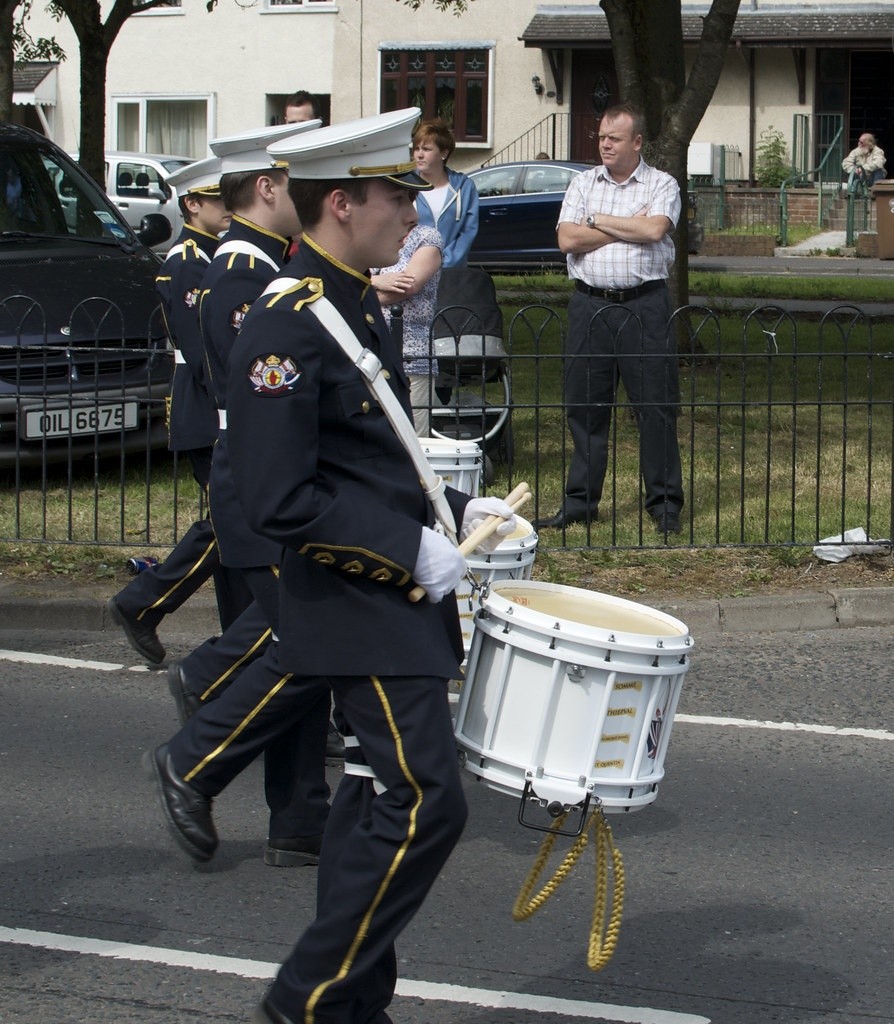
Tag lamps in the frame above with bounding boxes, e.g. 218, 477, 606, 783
532, 76, 542, 94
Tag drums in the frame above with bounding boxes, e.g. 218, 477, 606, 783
456, 578, 695, 814
420, 436, 482, 505
462, 509, 542, 652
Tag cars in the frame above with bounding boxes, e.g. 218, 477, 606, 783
0, 123, 176, 469
28, 155, 221, 258
459, 162, 599, 277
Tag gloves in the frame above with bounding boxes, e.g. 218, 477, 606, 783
459, 497, 512, 554
412, 526, 468, 604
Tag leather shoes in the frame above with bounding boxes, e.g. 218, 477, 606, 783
167, 661, 205, 728
325, 720, 346, 768
107, 596, 166, 665
264, 833, 324, 867
655, 513, 682, 535
531, 510, 598, 528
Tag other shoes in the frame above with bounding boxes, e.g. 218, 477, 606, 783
250, 997, 293, 1024
868, 190, 876, 199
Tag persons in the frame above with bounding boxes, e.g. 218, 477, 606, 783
841, 132, 887, 199
228, 106, 517, 1024
109, 89, 333, 867
530, 103, 686, 535
369, 188, 444, 437
529, 152, 549, 179
412, 119, 480, 268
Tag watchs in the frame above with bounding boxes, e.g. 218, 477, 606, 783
587, 213, 595, 229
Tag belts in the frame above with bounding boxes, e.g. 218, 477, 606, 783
142, 742, 218, 863
575, 279, 666, 303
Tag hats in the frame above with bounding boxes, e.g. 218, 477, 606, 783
164, 156, 223, 197
266, 107, 435, 191
208, 118, 322, 174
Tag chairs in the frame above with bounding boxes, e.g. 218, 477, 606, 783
118, 173, 134, 196
135, 173, 149, 197
